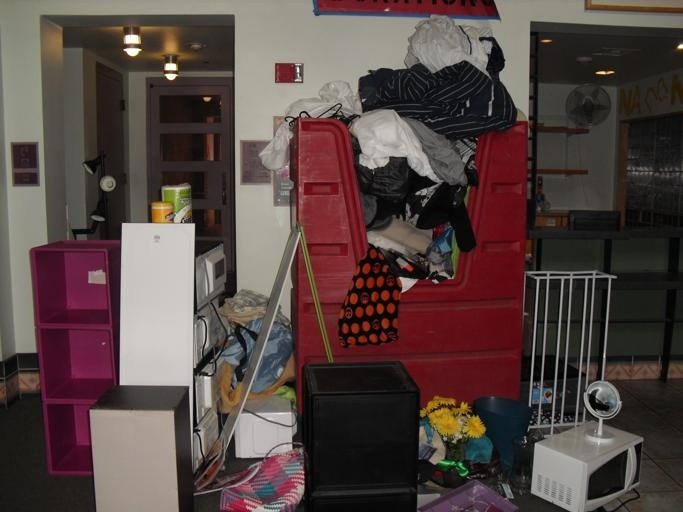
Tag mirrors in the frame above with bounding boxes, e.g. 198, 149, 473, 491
581, 381, 623, 443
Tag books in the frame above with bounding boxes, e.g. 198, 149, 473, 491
535, 208, 621, 231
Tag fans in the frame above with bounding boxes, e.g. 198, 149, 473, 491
561, 78, 612, 125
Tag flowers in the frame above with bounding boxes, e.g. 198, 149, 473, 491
419, 392, 487, 463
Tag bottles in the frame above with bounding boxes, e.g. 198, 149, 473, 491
151, 199, 174, 224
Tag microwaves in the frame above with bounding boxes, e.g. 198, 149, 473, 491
529, 420, 644, 512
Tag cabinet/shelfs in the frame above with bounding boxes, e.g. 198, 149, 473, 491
122, 223, 242, 477
287, 118, 530, 415
532, 225, 683, 389
28, 238, 120, 483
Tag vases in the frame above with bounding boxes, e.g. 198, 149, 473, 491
436, 458, 472, 490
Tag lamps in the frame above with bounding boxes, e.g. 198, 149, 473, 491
159, 51, 178, 82
65, 149, 116, 240
119, 22, 143, 58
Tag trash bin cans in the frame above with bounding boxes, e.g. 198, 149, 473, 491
470, 395, 530, 481
